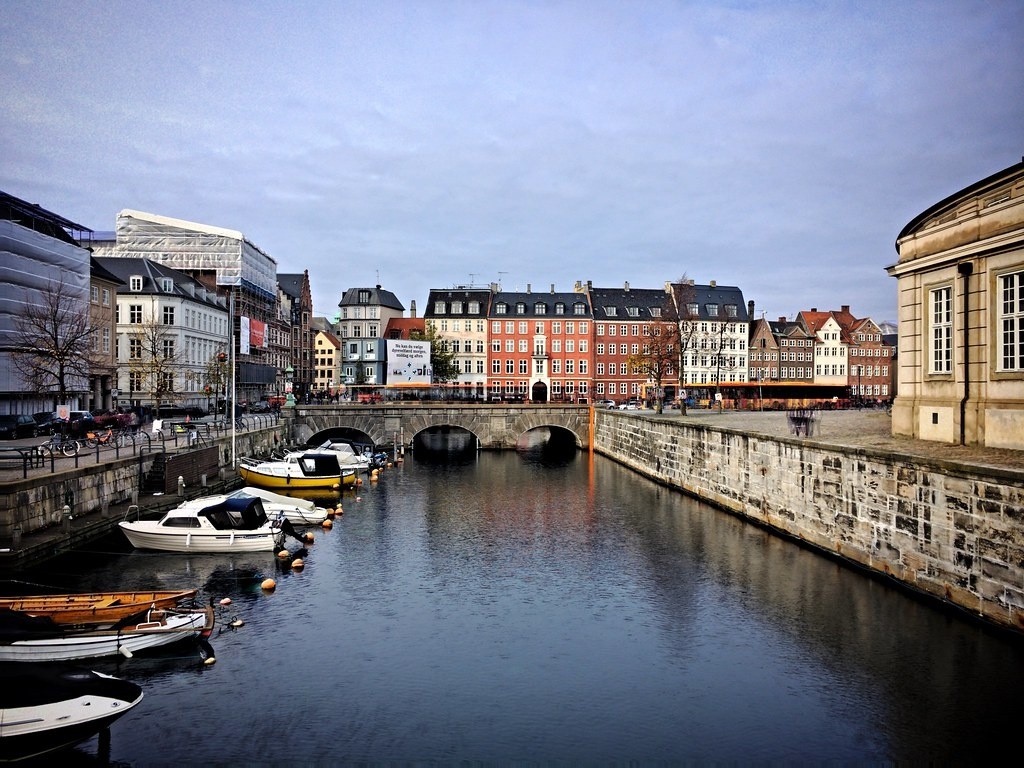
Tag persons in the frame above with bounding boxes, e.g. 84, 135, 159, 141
129, 410, 137, 435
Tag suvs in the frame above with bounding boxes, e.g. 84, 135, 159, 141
597, 400, 615, 409
0, 414, 39, 440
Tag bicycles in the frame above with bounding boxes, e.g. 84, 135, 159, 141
235, 418, 243, 433
38, 432, 80, 457
85, 425, 123, 448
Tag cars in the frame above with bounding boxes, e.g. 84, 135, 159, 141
92, 409, 132, 427
152, 403, 183, 417
63, 410, 95, 434
32, 412, 68, 436
618, 402, 640, 410
250, 400, 271, 412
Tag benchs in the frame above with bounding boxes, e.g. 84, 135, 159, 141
0, 450, 45, 468
90, 598, 120, 608
136, 622, 161, 630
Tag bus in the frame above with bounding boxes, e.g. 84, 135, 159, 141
358, 395, 382, 403
268, 396, 287, 406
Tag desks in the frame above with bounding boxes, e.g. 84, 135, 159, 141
0, 446, 39, 469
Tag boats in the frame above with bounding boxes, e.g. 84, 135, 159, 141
118, 495, 284, 552
239, 440, 372, 488
0, 611, 208, 665
0, 668, 145, 764
0, 596, 199, 626
177, 487, 329, 524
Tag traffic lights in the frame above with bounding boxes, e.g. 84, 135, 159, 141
205, 385, 209, 394
222, 385, 225, 395
219, 353, 228, 363
